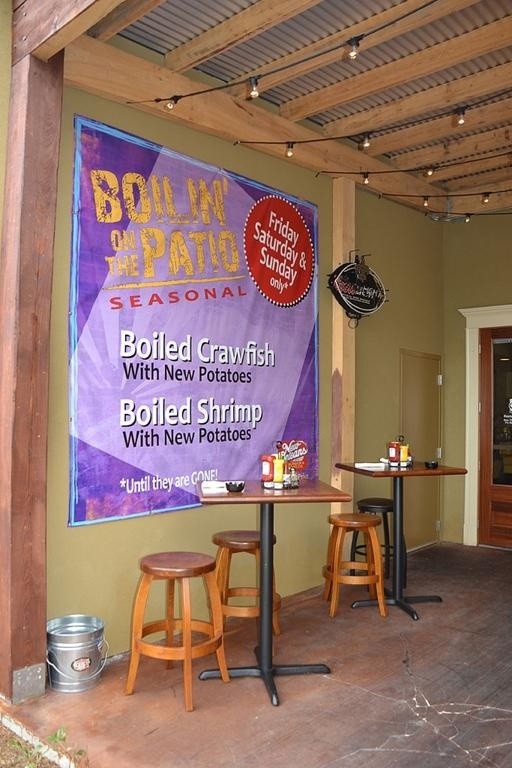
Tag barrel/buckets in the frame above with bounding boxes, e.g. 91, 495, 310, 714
46, 614, 109, 692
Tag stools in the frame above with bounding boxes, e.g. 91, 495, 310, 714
124, 530, 283, 715
323, 497, 408, 620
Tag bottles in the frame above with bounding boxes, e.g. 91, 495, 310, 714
261, 456, 300, 490
388, 442, 409, 467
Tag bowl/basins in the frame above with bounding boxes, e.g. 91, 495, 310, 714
425, 461, 438, 469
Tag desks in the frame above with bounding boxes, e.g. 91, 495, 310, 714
334, 460, 467, 623
193, 479, 352, 708
